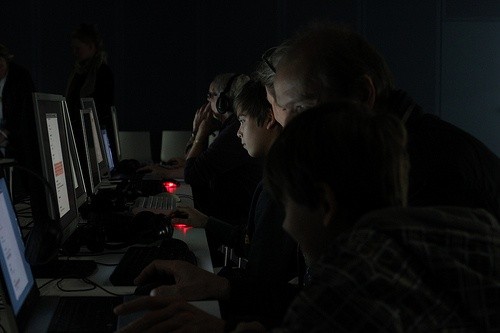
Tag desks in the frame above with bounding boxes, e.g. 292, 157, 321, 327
15, 164, 222, 333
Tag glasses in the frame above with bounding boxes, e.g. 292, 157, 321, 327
261, 46, 280, 74
207, 92, 220, 98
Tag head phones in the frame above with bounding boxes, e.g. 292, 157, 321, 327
104, 211, 174, 246
216, 72, 241, 114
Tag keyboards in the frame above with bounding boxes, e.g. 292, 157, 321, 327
136, 195, 177, 212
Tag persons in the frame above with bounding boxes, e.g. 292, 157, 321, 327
113, 38, 500, 333
68, 23, 114, 130
0, 44, 32, 147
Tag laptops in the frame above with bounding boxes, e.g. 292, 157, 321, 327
0, 165, 151, 333
101, 128, 114, 172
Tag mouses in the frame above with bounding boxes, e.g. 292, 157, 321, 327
167, 211, 188, 227
161, 178, 180, 188
134, 276, 175, 297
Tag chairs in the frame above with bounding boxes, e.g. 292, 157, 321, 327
119, 130, 219, 163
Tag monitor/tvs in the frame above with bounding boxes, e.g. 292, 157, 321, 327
62, 96, 88, 209
79, 97, 109, 177
79, 108, 102, 194
110, 106, 121, 156
31, 92, 80, 247
